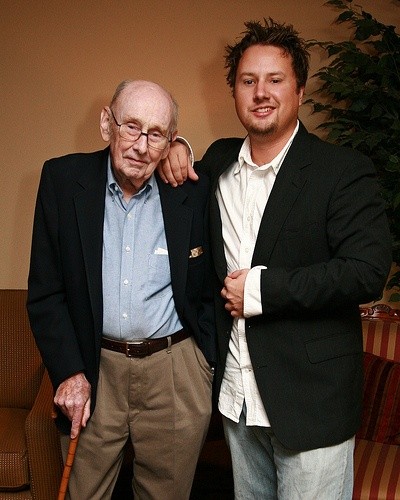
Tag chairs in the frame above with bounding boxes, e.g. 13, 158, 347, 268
0, 289, 67, 500
352, 304, 400, 500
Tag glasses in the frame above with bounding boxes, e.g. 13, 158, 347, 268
111, 109, 172, 150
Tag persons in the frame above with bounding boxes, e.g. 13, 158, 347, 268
158, 15, 396, 500
26, 80, 215, 500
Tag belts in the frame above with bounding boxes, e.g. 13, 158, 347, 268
102, 327, 191, 359
